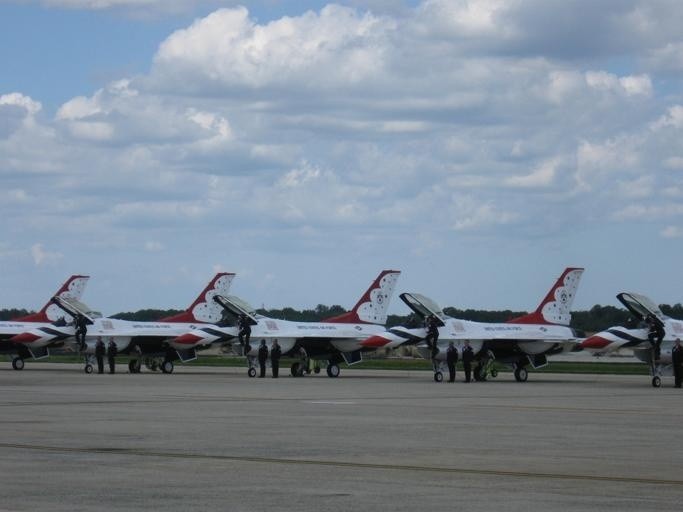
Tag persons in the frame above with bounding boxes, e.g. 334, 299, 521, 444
670, 338, 682, 388
444, 341, 458, 383
647, 312, 665, 362
237, 313, 251, 356
257, 338, 268, 378
107, 336, 116, 374
424, 315, 440, 359
297, 341, 313, 377
93, 335, 105, 373
74, 313, 88, 352
270, 338, 282, 378
461, 339, 474, 383
